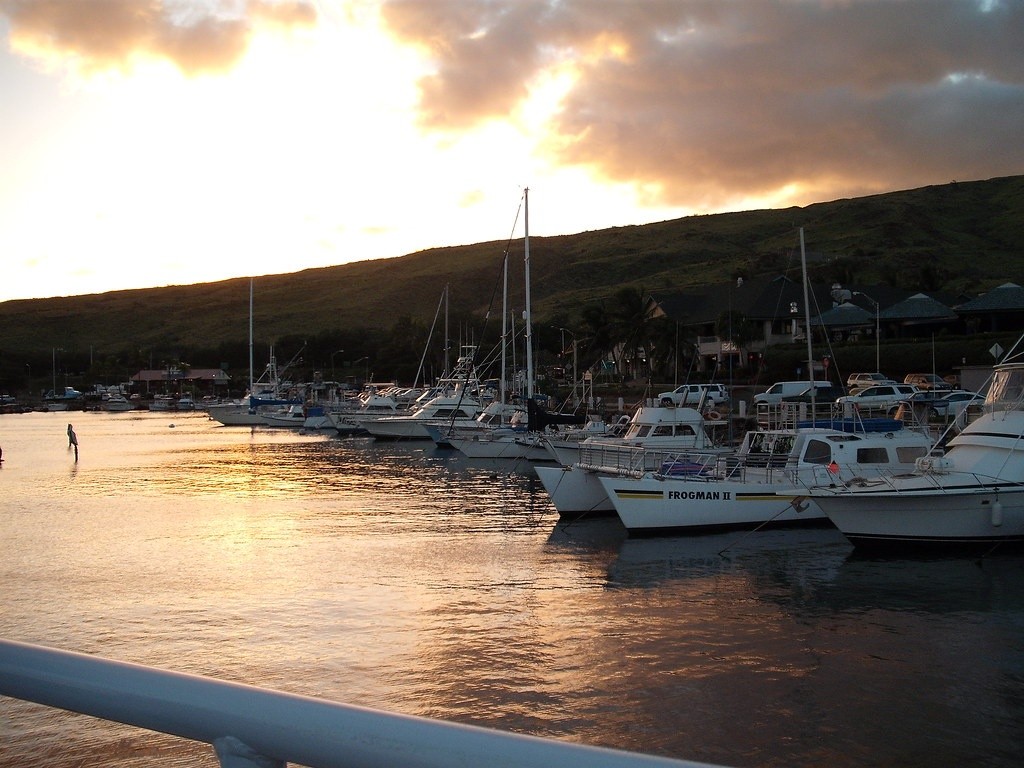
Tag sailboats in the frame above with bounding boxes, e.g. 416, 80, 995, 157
47, 348, 67, 410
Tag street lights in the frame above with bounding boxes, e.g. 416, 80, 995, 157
26, 363, 30, 394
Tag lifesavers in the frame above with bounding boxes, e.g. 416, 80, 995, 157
707, 410, 721, 420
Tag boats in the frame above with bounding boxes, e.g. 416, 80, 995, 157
203, 185, 1024, 557
176, 399, 196, 412
107, 402, 134, 411
149, 395, 175, 411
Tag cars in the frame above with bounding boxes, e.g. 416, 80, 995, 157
780, 386, 843, 415
929, 391, 986, 421
108, 394, 127, 402
130, 394, 140, 400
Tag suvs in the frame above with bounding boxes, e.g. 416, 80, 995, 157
658, 383, 729, 408
885, 388, 963, 420
903, 374, 954, 391
834, 384, 917, 413
847, 372, 897, 388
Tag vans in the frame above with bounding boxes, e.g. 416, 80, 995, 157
46, 387, 82, 400
753, 380, 831, 411
102, 393, 111, 400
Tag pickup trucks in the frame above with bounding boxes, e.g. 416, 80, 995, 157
0, 394, 15, 403
